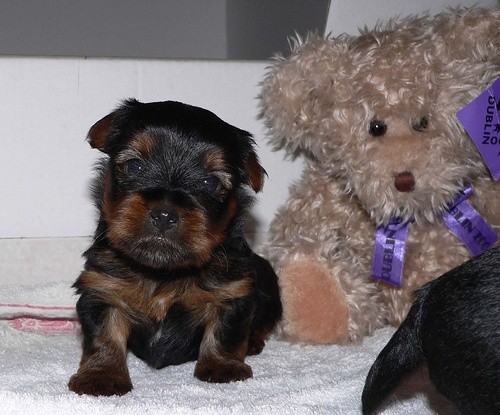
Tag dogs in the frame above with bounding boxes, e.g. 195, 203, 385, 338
360, 243, 500, 415
67, 97, 282, 398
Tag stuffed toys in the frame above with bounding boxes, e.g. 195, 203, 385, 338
256, 0, 500, 347
